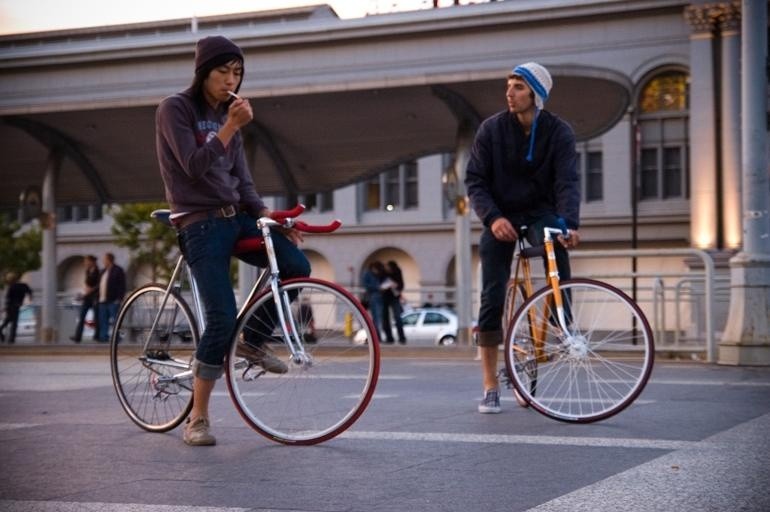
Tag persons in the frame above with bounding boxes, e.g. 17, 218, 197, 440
361, 260, 407, 346
68, 253, 126, 343
2, 270, 32, 346
465, 62, 581, 414
156, 37, 311, 445
297, 296, 318, 345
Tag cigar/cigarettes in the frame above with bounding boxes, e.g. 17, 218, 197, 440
227, 91, 242, 100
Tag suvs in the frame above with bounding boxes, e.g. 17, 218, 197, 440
0, 300, 114, 341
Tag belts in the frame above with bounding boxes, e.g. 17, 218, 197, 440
178, 204, 237, 229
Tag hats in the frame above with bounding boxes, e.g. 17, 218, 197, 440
512, 62, 554, 162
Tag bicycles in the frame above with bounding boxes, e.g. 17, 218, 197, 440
109, 202, 382, 447
501, 216, 656, 423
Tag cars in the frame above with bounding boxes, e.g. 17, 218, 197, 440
158, 308, 317, 344
352, 305, 479, 346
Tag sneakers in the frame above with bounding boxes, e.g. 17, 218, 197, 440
478, 389, 501, 413
183, 416, 216, 446
236, 341, 288, 373
